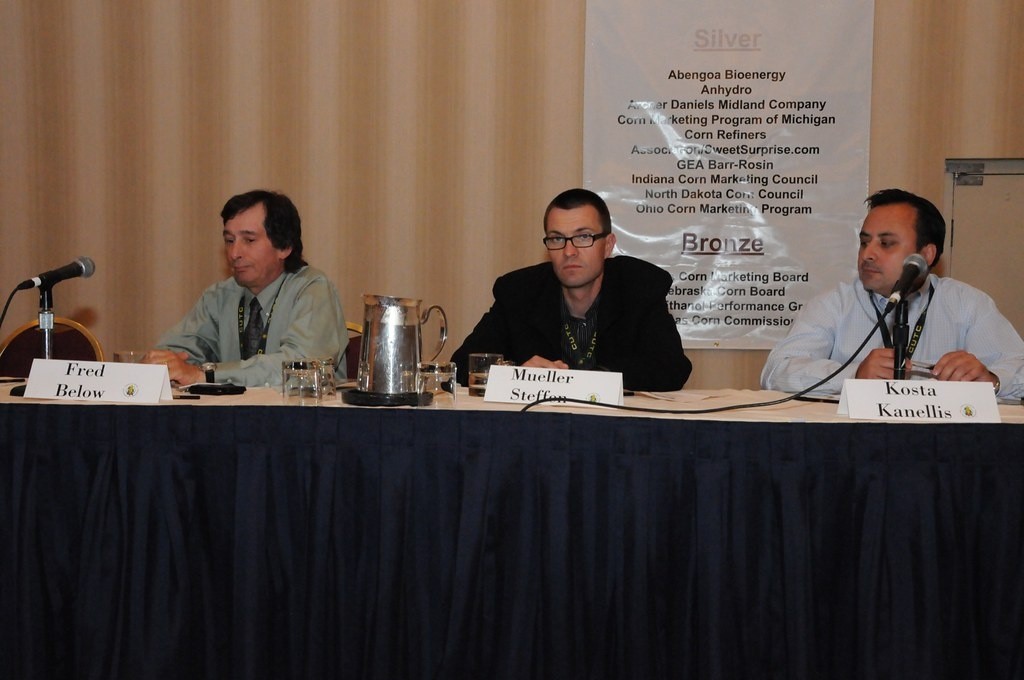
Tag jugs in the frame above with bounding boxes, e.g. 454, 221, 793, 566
356, 293, 448, 393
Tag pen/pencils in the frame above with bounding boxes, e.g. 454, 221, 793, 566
0, 379, 26, 383
624, 391, 634, 396
909, 359, 934, 369
173, 395, 200, 400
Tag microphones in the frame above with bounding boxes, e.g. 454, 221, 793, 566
18, 257, 97, 291
884, 253, 929, 313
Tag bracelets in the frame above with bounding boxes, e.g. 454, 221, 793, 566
993, 381, 1000, 395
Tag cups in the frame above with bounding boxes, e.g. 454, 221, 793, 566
416, 360, 456, 409
468, 353, 514, 398
281, 355, 337, 408
112, 350, 146, 363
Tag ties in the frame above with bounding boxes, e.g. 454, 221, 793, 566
242, 299, 264, 349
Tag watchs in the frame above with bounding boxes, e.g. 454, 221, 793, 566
199, 362, 217, 384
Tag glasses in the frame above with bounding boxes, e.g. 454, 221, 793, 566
542, 232, 603, 251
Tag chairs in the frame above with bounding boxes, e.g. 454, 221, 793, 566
0, 316, 104, 378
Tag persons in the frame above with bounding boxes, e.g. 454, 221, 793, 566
759, 189, 1024, 401
441, 188, 693, 394
138, 189, 348, 398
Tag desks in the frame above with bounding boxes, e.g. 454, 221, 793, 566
0, 376, 1024, 680
